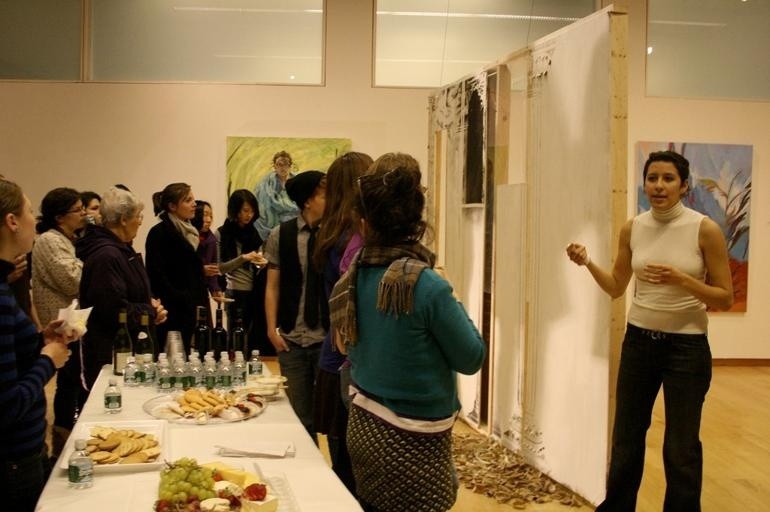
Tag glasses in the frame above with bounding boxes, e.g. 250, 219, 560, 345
125, 212, 144, 223
66, 205, 86, 214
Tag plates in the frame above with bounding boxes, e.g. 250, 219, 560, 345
61, 419, 168, 472
251, 258, 269, 264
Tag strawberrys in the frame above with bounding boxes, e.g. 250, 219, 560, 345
156, 500, 171, 512
187, 494, 197, 502
244, 483, 267, 500
210, 467, 222, 481
219, 487, 241, 507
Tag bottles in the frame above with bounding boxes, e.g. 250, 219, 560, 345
136, 310, 155, 364
103, 380, 122, 414
122, 352, 265, 388
69, 438, 93, 488
190, 306, 248, 357
111, 308, 134, 374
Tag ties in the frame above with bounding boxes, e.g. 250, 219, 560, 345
301, 226, 321, 334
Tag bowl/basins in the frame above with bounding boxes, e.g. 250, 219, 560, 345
247, 375, 287, 401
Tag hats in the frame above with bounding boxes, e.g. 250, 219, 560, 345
285, 170, 323, 210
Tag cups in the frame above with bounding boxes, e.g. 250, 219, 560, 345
210, 263, 222, 274
644, 260, 664, 283
163, 330, 186, 359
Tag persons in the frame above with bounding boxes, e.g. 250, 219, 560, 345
0, 148, 487, 512
565, 150, 733, 512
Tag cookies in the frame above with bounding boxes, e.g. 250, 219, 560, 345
85, 425, 160, 464
172, 387, 234, 419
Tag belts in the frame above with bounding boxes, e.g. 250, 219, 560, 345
629, 323, 707, 344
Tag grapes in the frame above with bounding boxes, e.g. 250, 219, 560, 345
159, 457, 216, 500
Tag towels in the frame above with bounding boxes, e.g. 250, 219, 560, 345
211, 437, 296, 459
